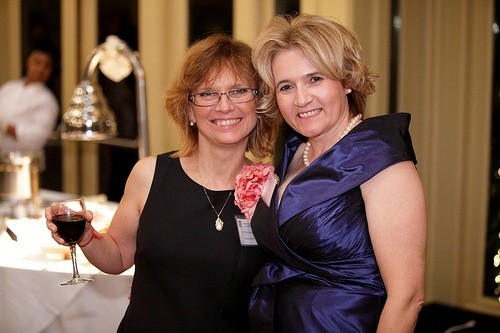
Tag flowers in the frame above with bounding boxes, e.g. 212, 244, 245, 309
232, 161, 275, 220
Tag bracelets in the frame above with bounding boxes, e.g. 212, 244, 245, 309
77, 227, 103, 248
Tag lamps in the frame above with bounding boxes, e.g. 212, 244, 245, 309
60, 41, 150, 160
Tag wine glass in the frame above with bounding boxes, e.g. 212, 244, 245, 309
49, 199, 95, 285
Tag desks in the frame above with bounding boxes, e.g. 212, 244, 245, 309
0, 188, 135, 333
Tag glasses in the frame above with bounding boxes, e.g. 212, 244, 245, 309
187, 87, 259, 107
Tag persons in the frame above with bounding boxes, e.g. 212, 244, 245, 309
0, 49, 60, 171
45, 34, 281, 333
251, 14, 428, 333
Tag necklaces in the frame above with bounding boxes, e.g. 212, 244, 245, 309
195, 155, 247, 232
302, 114, 362, 167
